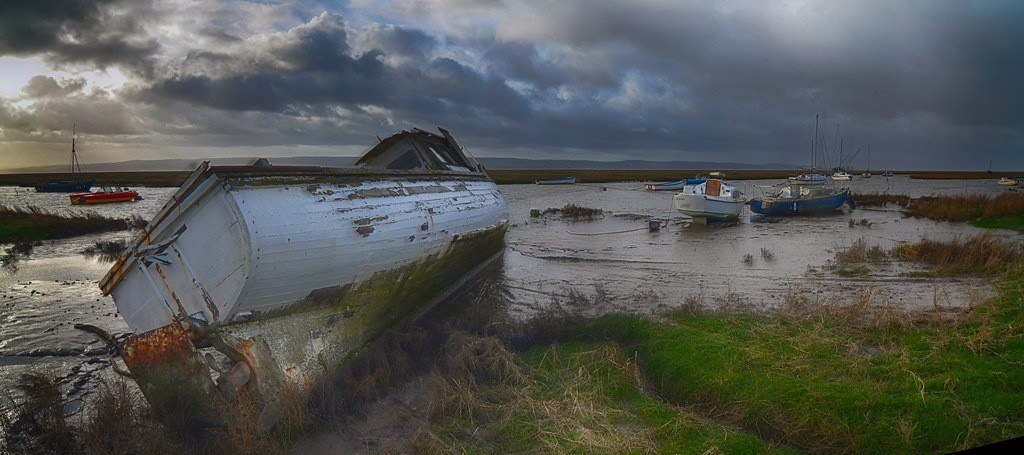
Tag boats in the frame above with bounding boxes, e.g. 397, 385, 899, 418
97, 121, 510, 455
33, 123, 92, 193
882, 171, 893, 176
746, 140, 851, 216
70, 183, 137, 203
673, 172, 746, 221
997, 178, 1019, 185
684, 176, 708, 184
645, 179, 687, 190
536, 175, 574, 185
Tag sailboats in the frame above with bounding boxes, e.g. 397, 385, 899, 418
831, 137, 853, 181
786, 113, 828, 185
861, 144, 872, 178
